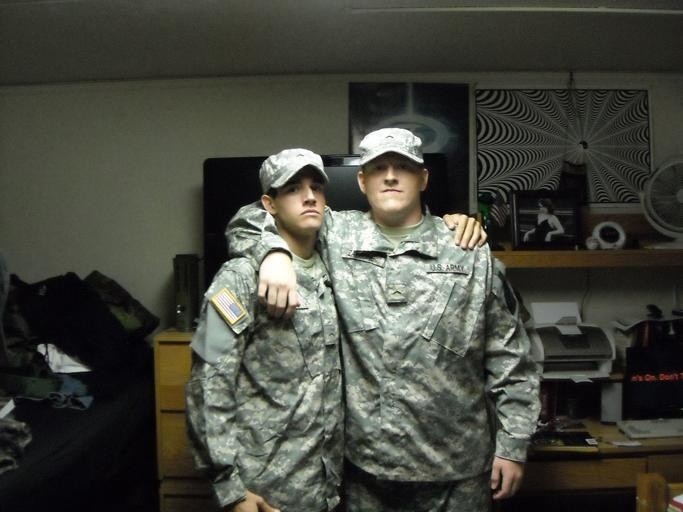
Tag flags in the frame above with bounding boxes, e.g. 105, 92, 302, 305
487, 195, 511, 228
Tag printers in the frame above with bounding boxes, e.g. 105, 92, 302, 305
527, 302, 616, 381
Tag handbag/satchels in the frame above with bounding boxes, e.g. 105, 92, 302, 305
622, 323, 683, 421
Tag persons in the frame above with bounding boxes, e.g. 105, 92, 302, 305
225, 127, 544, 512
523, 198, 565, 240
185, 146, 490, 512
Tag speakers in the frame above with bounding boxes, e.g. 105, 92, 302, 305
173, 254, 198, 332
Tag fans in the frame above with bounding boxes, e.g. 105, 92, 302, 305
639, 156, 683, 250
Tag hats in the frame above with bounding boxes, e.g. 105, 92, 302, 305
259, 148, 329, 195
358, 127, 424, 166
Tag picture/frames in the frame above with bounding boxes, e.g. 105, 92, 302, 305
347, 78, 468, 215
508, 187, 590, 251
469, 78, 658, 218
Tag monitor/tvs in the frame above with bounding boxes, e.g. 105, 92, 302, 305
625, 349, 683, 418
204, 156, 449, 297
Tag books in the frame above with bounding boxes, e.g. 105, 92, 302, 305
617, 418, 683, 440
529, 413, 601, 453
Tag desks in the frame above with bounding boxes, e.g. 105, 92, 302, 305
493, 419, 682, 512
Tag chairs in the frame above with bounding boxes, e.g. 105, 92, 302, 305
636, 470, 682, 512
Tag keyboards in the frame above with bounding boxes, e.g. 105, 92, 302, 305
619, 420, 682, 438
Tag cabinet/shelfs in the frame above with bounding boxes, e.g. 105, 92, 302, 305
145, 324, 222, 512
